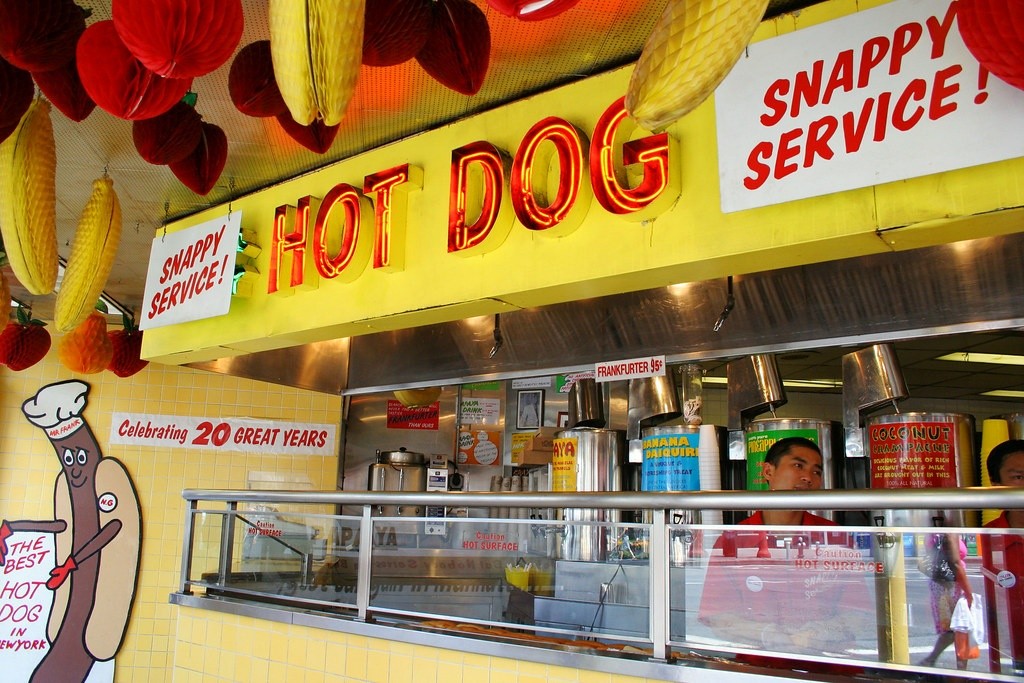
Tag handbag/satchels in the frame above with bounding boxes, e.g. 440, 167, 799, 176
918, 543, 956, 582
954, 631, 979, 658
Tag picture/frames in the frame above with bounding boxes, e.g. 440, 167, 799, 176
515, 389, 545, 430
556, 411, 569, 427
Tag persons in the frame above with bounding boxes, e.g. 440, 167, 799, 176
698, 436, 872, 650
918, 534, 974, 671
940, 438, 1024, 675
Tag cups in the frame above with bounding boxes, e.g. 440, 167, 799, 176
488, 475, 530, 550
533, 572, 552, 596
698, 424, 725, 569
980, 418, 1013, 528
505, 567, 529, 593
529, 571, 536, 585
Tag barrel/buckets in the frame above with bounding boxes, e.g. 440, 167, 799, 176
641, 425, 723, 567
551, 425, 623, 563
744, 417, 844, 524
866, 412, 980, 561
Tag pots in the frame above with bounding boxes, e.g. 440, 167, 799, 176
376, 447, 425, 467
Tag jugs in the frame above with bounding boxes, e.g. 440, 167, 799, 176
599, 583, 614, 603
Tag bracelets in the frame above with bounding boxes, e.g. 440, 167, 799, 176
761, 624, 782, 649
